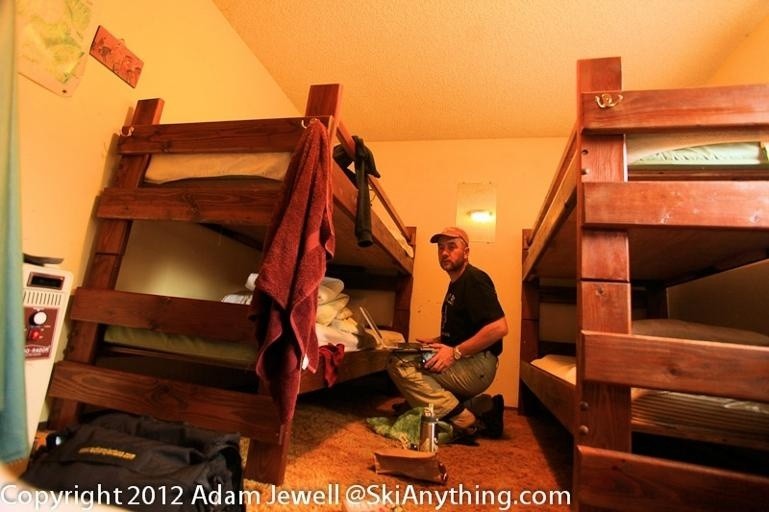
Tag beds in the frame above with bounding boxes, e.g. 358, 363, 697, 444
517, 58, 768, 507
47, 75, 417, 485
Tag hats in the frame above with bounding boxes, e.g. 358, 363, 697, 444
431, 227, 469, 246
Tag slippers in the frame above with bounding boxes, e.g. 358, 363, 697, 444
448, 395, 503, 443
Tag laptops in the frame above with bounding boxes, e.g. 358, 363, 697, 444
359, 306, 435, 353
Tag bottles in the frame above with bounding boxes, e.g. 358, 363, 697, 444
418, 405, 436, 454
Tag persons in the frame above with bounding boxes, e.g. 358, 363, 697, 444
387, 227, 509, 444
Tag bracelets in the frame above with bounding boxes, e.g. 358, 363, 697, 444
454, 345, 462, 361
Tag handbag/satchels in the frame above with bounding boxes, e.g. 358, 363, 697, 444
17, 412, 246, 512
